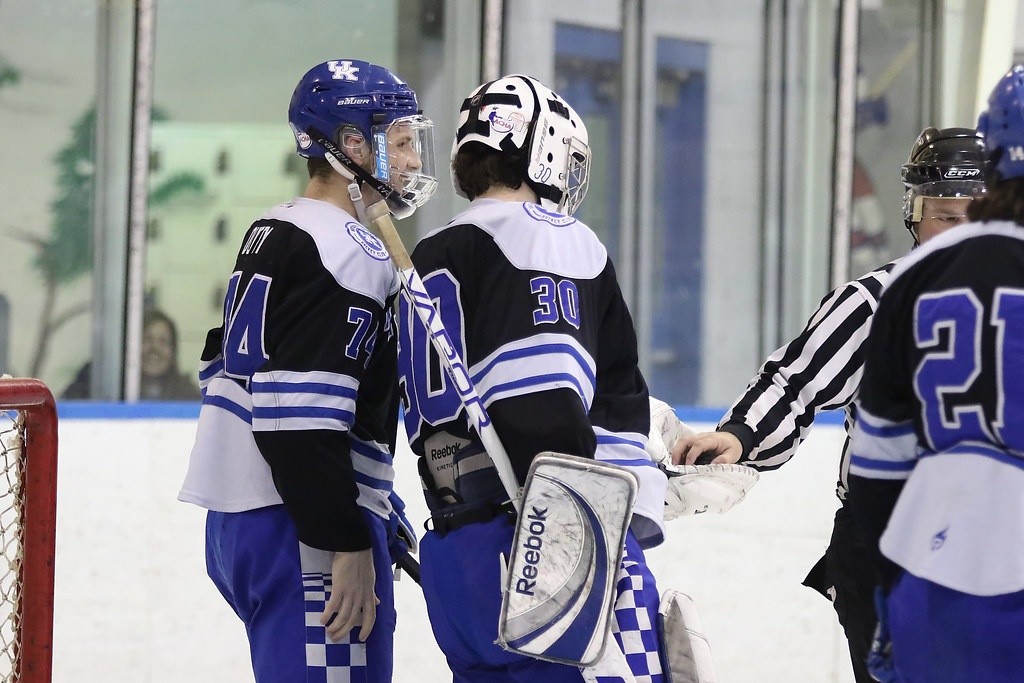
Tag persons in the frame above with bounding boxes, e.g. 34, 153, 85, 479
57, 58, 762, 683
671, 64, 1024, 682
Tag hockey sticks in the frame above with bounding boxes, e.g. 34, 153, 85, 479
366, 198, 522, 514
395, 552, 423, 588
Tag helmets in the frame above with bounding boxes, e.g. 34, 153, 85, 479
288, 58, 439, 221
977, 62, 1024, 180
903, 126, 987, 224
451, 73, 591, 217
